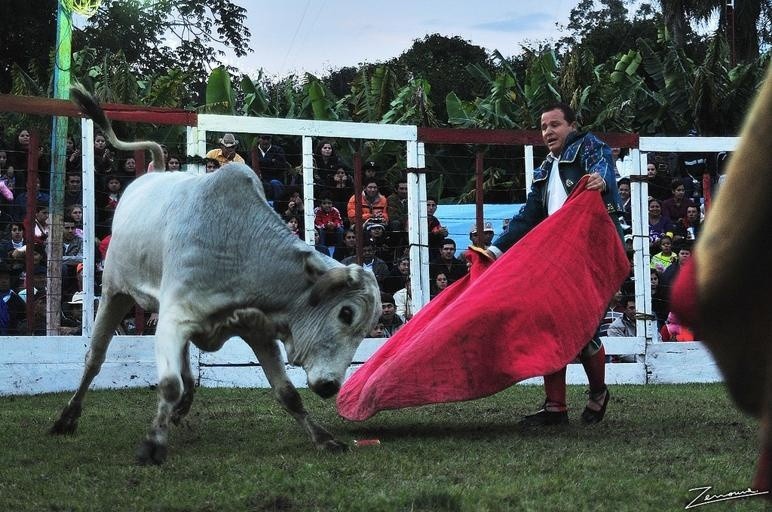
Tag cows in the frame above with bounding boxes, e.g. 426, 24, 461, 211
45, 76, 383, 466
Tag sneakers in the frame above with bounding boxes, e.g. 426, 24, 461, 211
525, 398, 570, 426
580, 384, 610, 426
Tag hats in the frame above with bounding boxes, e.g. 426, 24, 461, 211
67, 290, 99, 306
470, 222, 478, 234
219, 133, 240, 148
77, 262, 83, 273
483, 220, 494, 232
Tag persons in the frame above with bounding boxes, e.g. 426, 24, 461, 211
670, 55, 770, 500
487, 103, 628, 426
